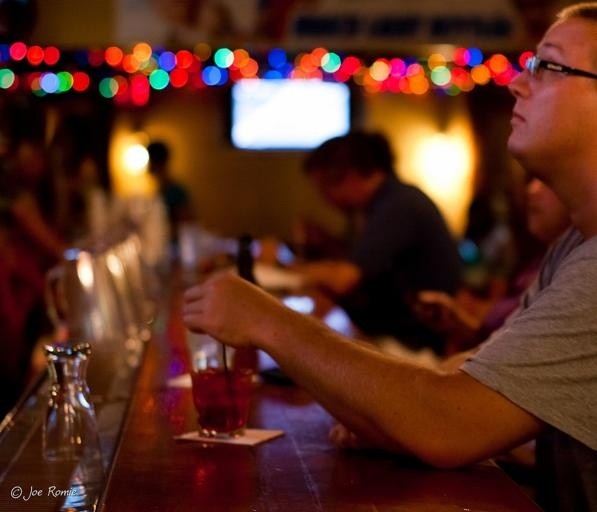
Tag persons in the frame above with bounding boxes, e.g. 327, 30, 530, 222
287, 126, 459, 351
458, 170, 586, 353
292, 134, 365, 254
180, 3, 595, 512
0, 108, 106, 422
148, 139, 197, 223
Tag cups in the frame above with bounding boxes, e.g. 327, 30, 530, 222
188, 366, 258, 439
39, 341, 105, 464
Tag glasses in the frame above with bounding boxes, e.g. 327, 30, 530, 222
526, 56, 597, 79
